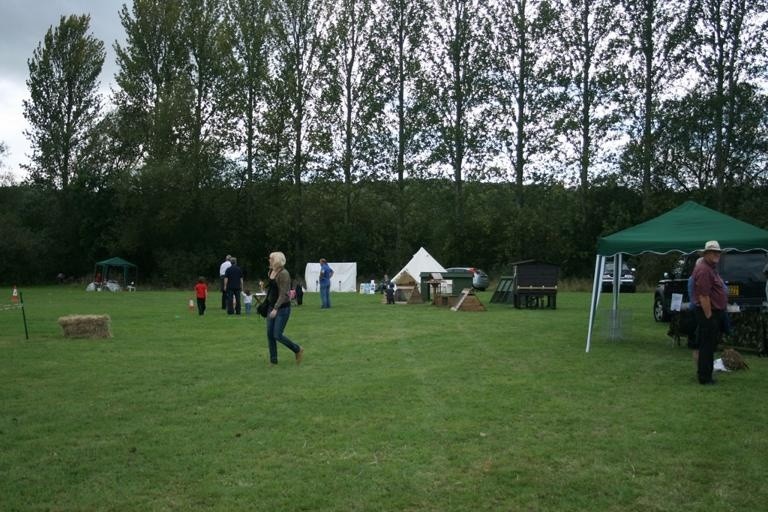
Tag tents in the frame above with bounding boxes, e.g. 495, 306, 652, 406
389, 246, 453, 295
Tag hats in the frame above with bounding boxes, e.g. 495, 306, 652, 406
696, 241, 724, 253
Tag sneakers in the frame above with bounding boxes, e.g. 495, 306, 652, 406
295, 346, 303, 364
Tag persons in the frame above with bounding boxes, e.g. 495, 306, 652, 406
241, 290, 254, 317
318, 258, 335, 310
219, 254, 233, 310
384, 284, 396, 304
686, 257, 705, 358
223, 257, 244, 316
194, 276, 209, 317
265, 251, 305, 368
689, 240, 730, 386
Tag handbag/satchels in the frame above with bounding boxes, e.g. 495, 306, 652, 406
256, 299, 268, 317
719, 349, 749, 370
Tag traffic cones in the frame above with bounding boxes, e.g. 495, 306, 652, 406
8, 286, 20, 307
186, 296, 195, 313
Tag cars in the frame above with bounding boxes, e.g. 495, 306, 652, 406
600, 259, 639, 295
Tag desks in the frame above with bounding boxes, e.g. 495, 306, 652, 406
252, 293, 268, 306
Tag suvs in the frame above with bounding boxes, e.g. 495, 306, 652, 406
650, 246, 768, 326
442, 266, 489, 290
